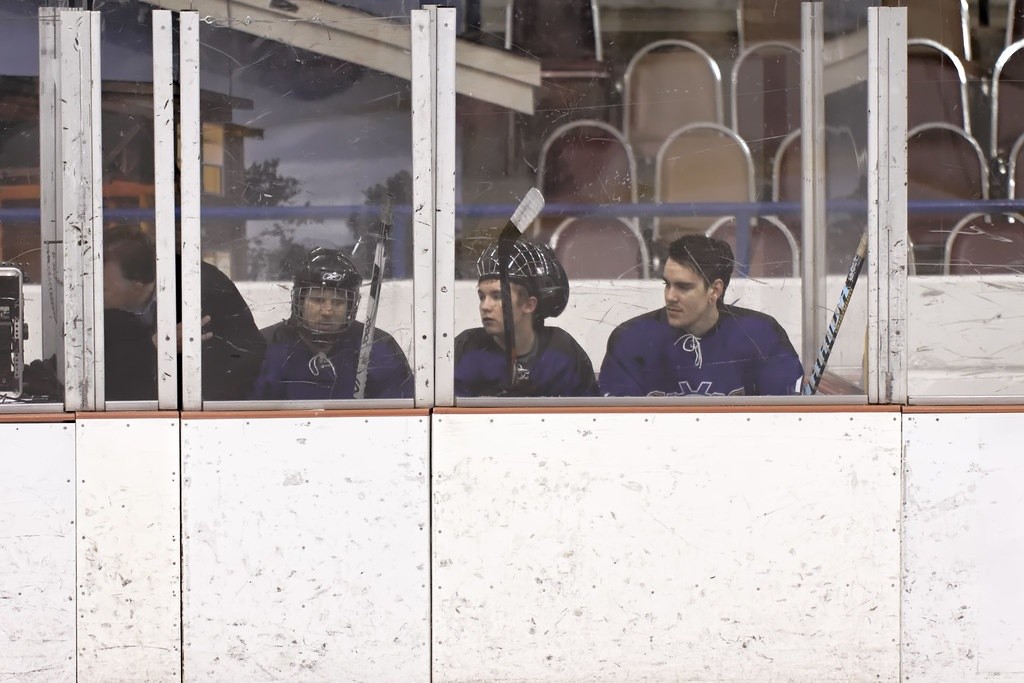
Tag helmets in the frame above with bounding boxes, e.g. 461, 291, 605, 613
476, 239, 569, 318
290, 247, 363, 346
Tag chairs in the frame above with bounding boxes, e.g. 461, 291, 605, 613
514, 0, 1023, 280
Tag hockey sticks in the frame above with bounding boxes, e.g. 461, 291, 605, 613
351, 192, 395, 400
497, 186, 546, 398
803, 226, 867, 396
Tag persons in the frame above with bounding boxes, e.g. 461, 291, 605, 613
249, 248, 415, 401
454, 238, 600, 397
103, 223, 267, 402
597, 235, 805, 397
